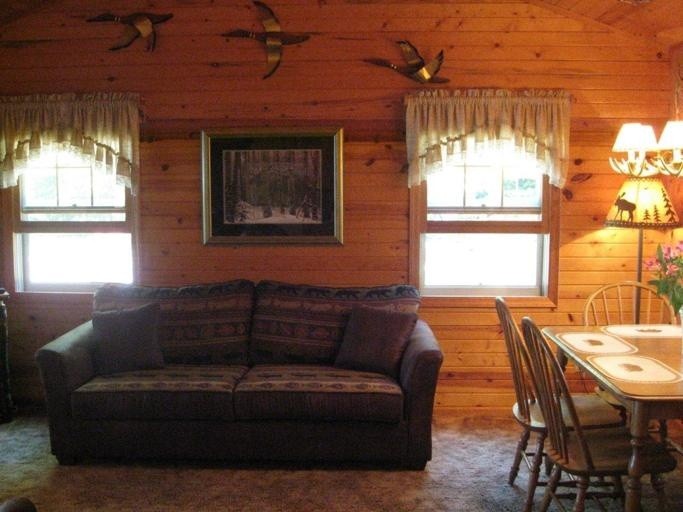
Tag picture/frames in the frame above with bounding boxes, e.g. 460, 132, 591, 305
198, 124, 348, 248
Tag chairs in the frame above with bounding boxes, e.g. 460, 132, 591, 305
517, 314, 677, 511
491, 295, 627, 512
580, 278, 679, 454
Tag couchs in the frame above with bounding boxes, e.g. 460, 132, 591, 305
31, 275, 447, 474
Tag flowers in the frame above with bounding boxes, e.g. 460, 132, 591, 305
639, 236, 683, 315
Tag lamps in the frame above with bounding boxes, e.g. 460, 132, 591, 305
602, 175, 679, 325
598, 118, 683, 183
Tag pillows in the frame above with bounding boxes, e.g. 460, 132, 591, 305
329, 298, 421, 379
89, 301, 167, 377
